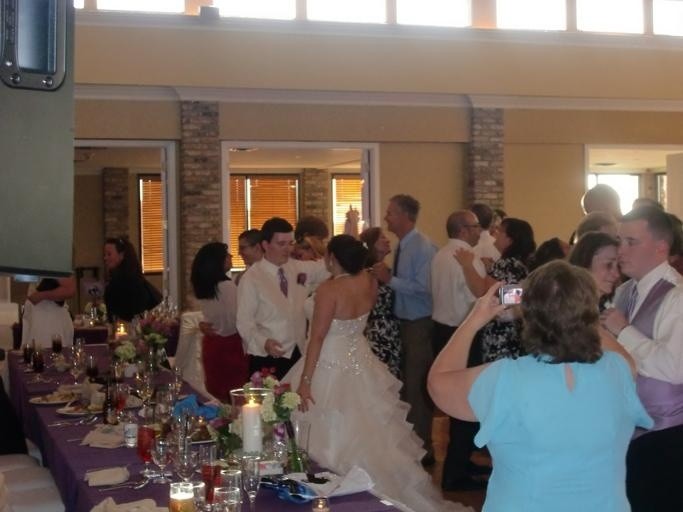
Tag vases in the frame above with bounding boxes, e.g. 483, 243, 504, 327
201, 445, 231, 507
282, 421, 304, 473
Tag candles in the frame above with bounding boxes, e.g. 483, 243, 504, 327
241, 399, 263, 453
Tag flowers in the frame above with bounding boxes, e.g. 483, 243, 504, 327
243, 363, 302, 472
108, 309, 173, 417
83, 300, 107, 320
204, 401, 243, 503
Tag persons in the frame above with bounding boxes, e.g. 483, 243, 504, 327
102, 238, 163, 324
534, 184, 682, 511
190, 205, 477, 511
427, 259, 654, 511
21, 276, 76, 349
359, 193, 537, 491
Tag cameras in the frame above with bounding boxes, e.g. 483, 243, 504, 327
500, 285, 526, 307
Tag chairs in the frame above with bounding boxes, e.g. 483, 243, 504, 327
0, 452, 66, 512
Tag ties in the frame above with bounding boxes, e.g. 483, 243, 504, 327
279, 268, 287, 297
391, 244, 400, 312
627, 285, 639, 317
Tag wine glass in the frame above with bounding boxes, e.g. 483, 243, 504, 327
141, 297, 177, 326
72, 306, 106, 327
22, 334, 333, 511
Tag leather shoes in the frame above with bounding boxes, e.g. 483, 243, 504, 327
443, 474, 488, 490
421, 455, 435, 467
469, 460, 492, 473
